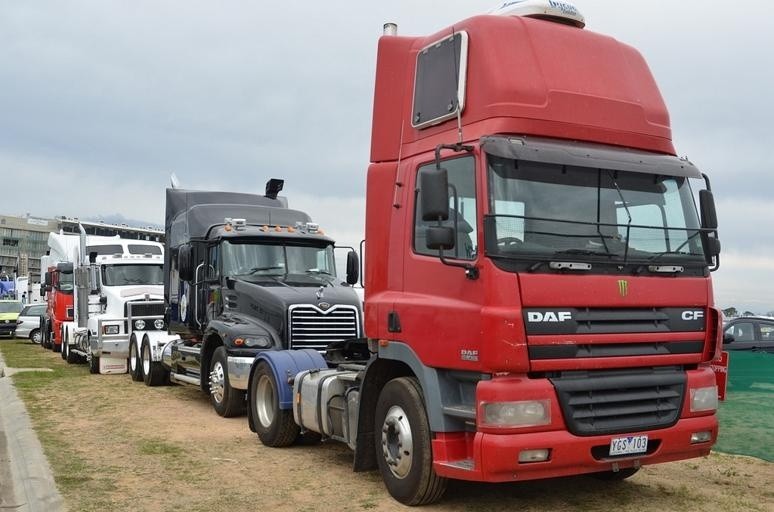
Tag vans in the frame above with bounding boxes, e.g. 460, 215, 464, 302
11, 304, 50, 343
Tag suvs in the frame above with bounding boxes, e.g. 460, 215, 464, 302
0, 300, 26, 334
721, 313, 774, 388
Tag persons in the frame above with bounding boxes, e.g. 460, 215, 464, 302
22, 291, 26, 304
